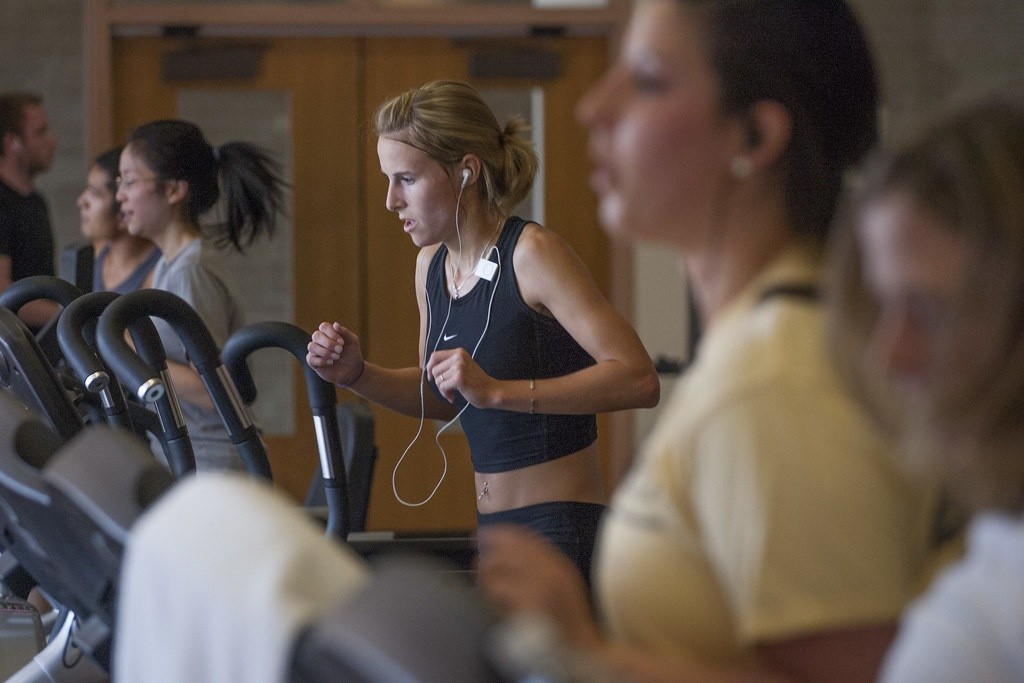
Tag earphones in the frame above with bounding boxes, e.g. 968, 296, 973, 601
460, 169, 472, 189
741, 121, 761, 148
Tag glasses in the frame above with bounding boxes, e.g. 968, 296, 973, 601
115, 176, 165, 190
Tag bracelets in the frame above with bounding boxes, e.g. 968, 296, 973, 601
337, 359, 366, 388
527, 379, 536, 414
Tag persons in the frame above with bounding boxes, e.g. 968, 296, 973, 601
0, 0, 1024, 683
305, 83, 659, 584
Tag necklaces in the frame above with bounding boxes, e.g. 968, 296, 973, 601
450, 213, 505, 301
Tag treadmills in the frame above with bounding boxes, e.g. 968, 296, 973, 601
1, 271, 483, 683
